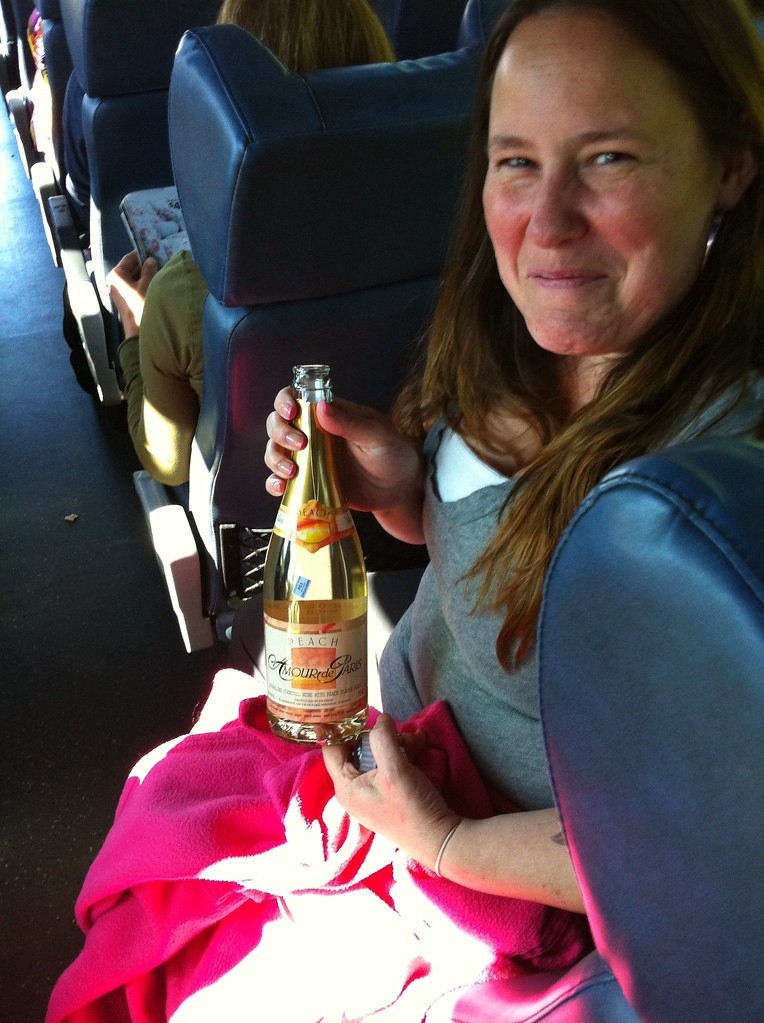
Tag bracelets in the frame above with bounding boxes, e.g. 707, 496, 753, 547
434, 818, 461, 878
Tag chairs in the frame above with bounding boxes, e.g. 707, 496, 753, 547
0, 0, 764, 1023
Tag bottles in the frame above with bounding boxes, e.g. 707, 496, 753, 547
262, 364, 367, 743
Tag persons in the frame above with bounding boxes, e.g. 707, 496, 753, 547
47, 0, 764, 1021
104, 1, 407, 486
